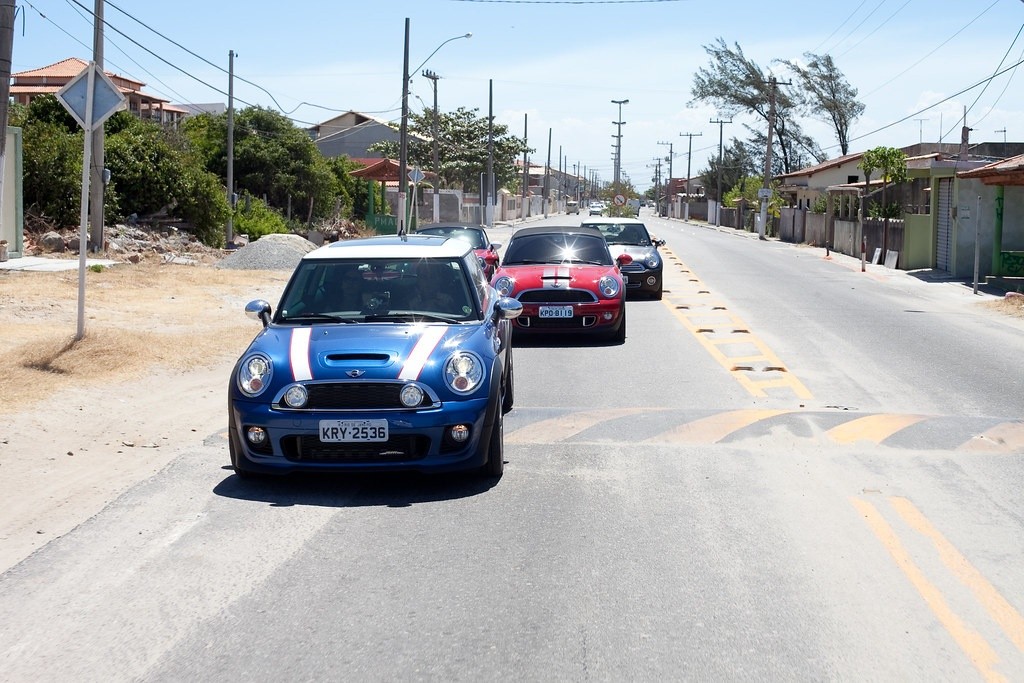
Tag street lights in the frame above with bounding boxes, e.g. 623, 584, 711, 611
610, 99, 629, 169
398, 17, 474, 228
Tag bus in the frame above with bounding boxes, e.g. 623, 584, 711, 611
566, 200, 581, 215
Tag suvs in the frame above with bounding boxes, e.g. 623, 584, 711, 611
648, 200, 654, 209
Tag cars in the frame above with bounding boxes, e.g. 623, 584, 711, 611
224, 233, 525, 481
640, 200, 646, 207
580, 217, 666, 300
588, 206, 604, 217
590, 201, 611, 209
414, 222, 500, 278
488, 225, 626, 343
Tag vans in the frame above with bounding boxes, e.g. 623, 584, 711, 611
626, 198, 640, 216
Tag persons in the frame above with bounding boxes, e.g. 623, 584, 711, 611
319, 267, 373, 314
406, 269, 456, 314
